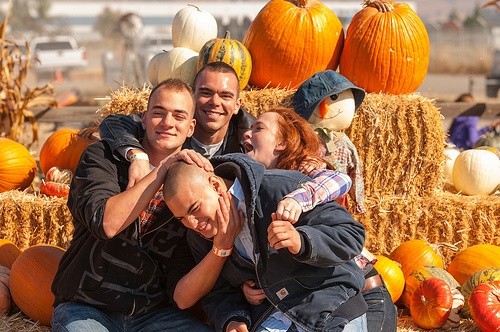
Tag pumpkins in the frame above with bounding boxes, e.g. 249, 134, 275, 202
39, 127, 101, 197
0, 239, 67, 327
372, 239, 500, 332
242, 0, 344, 90
339, 0, 431, 95
444, 146, 500, 196
147, 4, 252, 92
0, 137, 37, 193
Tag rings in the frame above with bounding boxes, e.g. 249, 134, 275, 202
284, 208, 290, 213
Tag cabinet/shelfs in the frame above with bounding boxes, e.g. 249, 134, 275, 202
0, 83, 500, 332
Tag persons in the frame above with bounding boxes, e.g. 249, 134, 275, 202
163, 153, 397, 332
52, 78, 245, 332
243, 107, 352, 303
290, 68, 366, 215
99, 62, 257, 191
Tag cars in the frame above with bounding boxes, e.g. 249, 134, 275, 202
2, 37, 28, 72
99, 29, 175, 78
26, 35, 88, 80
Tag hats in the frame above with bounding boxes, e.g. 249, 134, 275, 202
292, 71, 369, 124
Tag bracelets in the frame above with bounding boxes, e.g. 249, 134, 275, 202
212, 245, 233, 256
130, 153, 149, 163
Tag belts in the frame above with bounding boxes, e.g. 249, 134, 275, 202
362, 272, 386, 296
